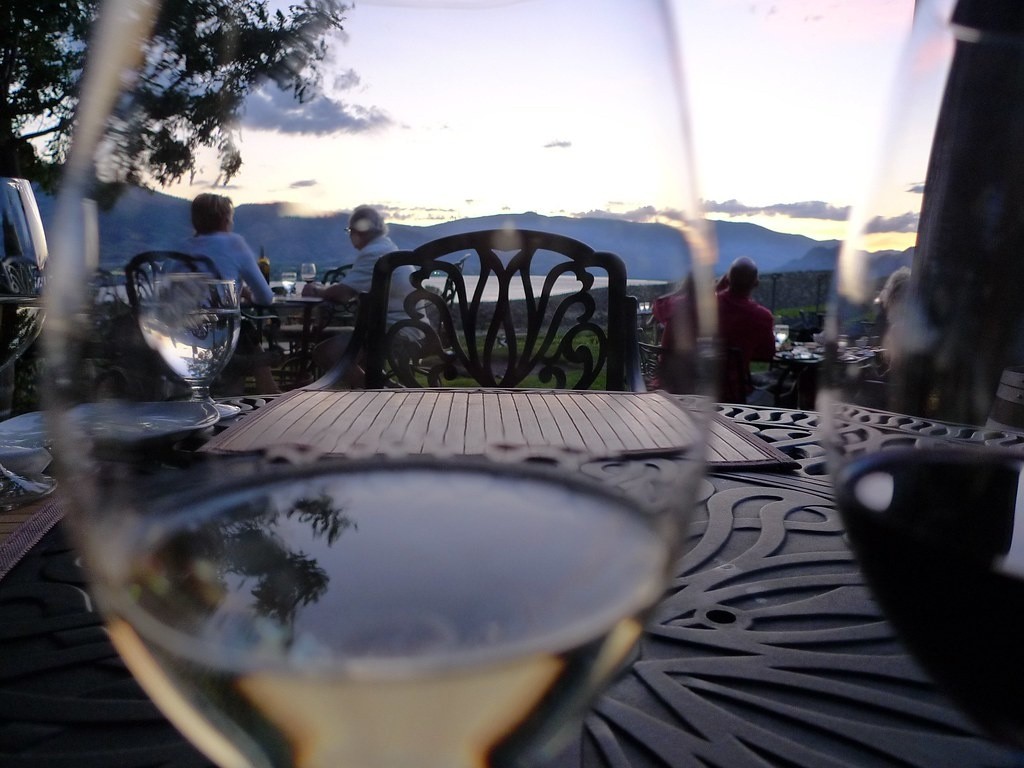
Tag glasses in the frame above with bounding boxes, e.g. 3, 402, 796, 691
344, 227, 352, 232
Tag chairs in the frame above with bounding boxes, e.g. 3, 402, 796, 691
302, 227, 646, 393
121, 250, 284, 363
385, 251, 474, 386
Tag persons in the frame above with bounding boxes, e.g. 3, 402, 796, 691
301, 206, 431, 388
653, 253, 778, 405
161, 192, 281, 396
874, 268, 911, 335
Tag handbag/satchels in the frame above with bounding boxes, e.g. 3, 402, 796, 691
781, 310, 823, 342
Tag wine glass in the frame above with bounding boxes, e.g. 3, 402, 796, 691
281, 273, 297, 300
153, 270, 242, 419
774, 324, 790, 345
300, 262, 316, 284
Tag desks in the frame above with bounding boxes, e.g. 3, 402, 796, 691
776, 339, 883, 380
241, 295, 322, 377
0, 386, 1024, 767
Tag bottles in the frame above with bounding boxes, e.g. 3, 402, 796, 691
257, 245, 270, 286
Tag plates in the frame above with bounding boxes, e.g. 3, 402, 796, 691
0, 398, 220, 455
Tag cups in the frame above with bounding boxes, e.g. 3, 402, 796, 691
837, 334, 848, 349
36, 1, 722, 768
645, 301, 649, 310
639, 302, 645, 311
812, 0, 1024, 749
0, 176, 49, 372
